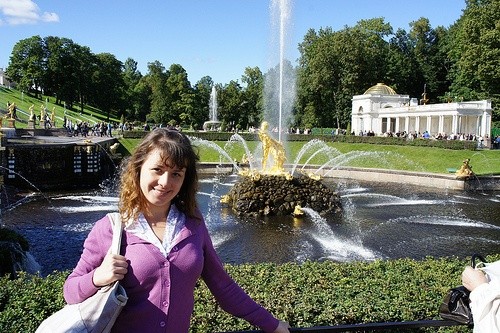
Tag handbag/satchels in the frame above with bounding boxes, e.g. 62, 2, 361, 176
438, 254, 488, 325
35, 211, 129, 333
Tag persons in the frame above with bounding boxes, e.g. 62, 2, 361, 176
478, 135, 483, 147
166, 124, 182, 131
384, 131, 476, 141
486, 134, 500, 149
249, 127, 311, 134
461, 261, 500, 333
152, 123, 159, 130
6, 101, 18, 119
63, 116, 113, 137
63, 129, 291, 333
331, 128, 374, 137
33, 114, 52, 128
228, 121, 238, 132
113, 121, 150, 131
